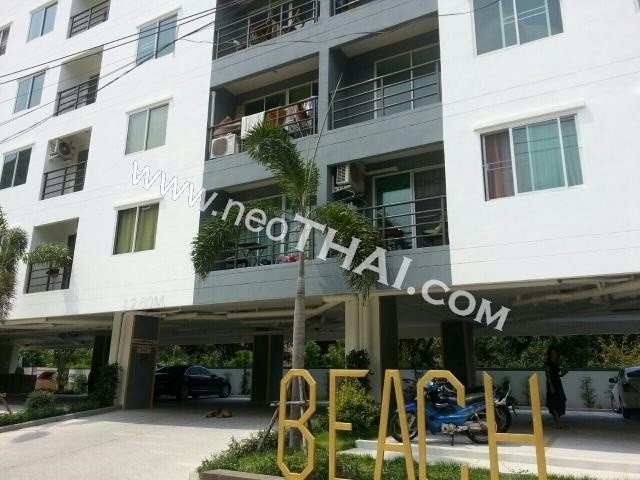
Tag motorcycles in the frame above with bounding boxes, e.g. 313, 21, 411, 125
388, 369, 518, 447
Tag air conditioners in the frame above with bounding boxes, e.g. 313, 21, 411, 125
336, 160, 366, 194
209, 134, 239, 160
49, 139, 73, 160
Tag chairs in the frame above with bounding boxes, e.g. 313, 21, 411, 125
222, 241, 248, 269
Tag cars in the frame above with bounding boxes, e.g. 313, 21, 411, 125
155, 364, 232, 402
608, 366, 640, 419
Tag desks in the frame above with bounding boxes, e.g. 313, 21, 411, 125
238, 241, 268, 266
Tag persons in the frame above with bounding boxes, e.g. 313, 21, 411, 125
544, 347, 569, 429
